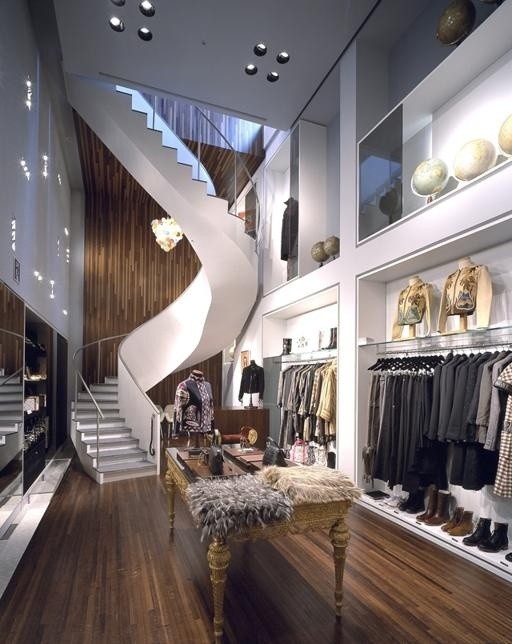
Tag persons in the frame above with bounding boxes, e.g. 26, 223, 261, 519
435, 255, 492, 335
175, 368, 215, 435
392, 276, 435, 340
236, 358, 265, 409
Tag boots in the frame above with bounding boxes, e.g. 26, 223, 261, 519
478, 523, 508, 553
441, 507, 473, 536
415, 488, 451, 526
399, 487, 425, 513
463, 518, 491, 545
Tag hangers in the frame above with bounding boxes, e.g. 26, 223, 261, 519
367, 338, 512, 378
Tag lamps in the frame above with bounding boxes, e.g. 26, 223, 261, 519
152, 215, 183, 251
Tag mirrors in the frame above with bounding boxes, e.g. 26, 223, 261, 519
0, 281, 24, 527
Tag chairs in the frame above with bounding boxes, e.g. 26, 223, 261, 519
155, 405, 190, 440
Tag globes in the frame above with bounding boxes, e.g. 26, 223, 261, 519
311, 236, 340, 268
496, 112, 511, 158
436, 0, 475, 46
450, 136, 496, 184
411, 157, 449, 206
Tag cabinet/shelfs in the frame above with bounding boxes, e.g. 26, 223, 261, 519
359, 1, 511, 578
23, 302, 53, 497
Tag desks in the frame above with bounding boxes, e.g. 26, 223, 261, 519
165, 445, 354, 644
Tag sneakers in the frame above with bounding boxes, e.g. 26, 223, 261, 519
383, 495, 407, 507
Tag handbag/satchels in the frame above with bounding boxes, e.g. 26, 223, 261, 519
199, 446, 210, 464
290, 439, 336, 469
208, 446, 224, 475
262, 436, 288, 467
